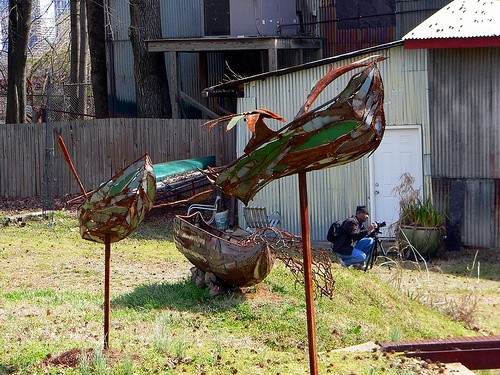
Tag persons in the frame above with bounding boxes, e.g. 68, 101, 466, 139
332, 206, 378, 270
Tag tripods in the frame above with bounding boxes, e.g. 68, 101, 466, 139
363, 236, 391, 273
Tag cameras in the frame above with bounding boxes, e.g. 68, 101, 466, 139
374, 221, 386, 232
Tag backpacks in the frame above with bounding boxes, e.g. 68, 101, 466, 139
327, 217, 359, 247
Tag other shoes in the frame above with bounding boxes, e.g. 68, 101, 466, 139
339, 258, 348, 269
355, 265, 370, 270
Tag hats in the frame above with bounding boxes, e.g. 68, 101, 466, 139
356, 206, 369, 216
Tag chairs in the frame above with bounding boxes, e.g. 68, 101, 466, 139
242, 206, 281, 239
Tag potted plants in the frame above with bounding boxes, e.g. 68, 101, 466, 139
397, 192, 447, 262
